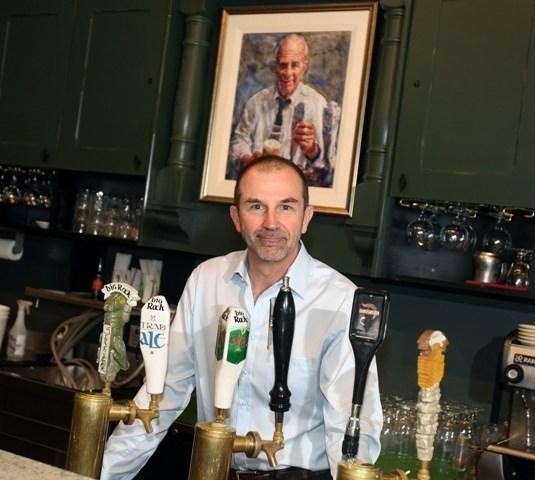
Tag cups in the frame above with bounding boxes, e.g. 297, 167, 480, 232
472, 251, 508, 283
520, 407, 535, 448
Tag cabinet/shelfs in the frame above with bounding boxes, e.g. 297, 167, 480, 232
354, 1, 535, 208
0, 0, 194, 206
1, 286, 196, 480
13, 196, 535, 306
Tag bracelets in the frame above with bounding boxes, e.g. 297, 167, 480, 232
303, 140, 321, 162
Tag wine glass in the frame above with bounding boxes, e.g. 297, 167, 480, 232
408, 203, 479, 252
380, 391, 485, 480
481, 423, 502, 450
507, 246, 534, 289
0, 165, 52, 208
262, 125, 285, 157
482, 212, 513, 254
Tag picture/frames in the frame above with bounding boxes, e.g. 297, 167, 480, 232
198, 0, 380, 220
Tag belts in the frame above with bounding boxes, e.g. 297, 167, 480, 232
226, 466, 331, 480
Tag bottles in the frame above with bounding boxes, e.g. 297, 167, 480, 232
91, 259, 104, 299
75, 188, 144, 241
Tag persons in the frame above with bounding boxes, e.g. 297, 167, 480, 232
100, 155, 383, 480
229, 33, 333, 183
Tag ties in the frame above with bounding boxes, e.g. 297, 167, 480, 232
267, 96, 291, 142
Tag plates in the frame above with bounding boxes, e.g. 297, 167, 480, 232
515, 323, 534, 346
36, 221, 49, 229
